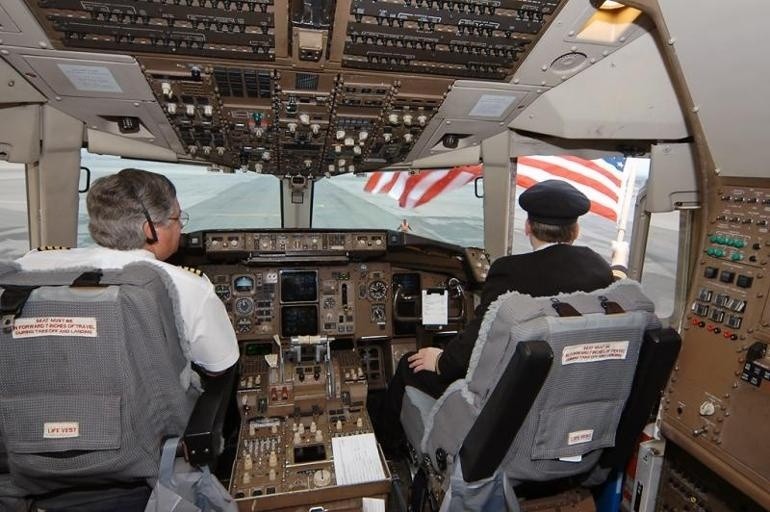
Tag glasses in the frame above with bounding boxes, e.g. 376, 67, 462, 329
169, 210, 190, 228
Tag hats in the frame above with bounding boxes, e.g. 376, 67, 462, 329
519, 180, 590, 225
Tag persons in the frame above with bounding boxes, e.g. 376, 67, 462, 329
377, 179, 632, 463
13, 165, 242, 468
396, 219, 413, 232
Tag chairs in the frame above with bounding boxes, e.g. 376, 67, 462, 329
0, 259, 240, 511
398, 278, 682, 511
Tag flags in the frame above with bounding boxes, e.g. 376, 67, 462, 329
363, 154, 629, 224
